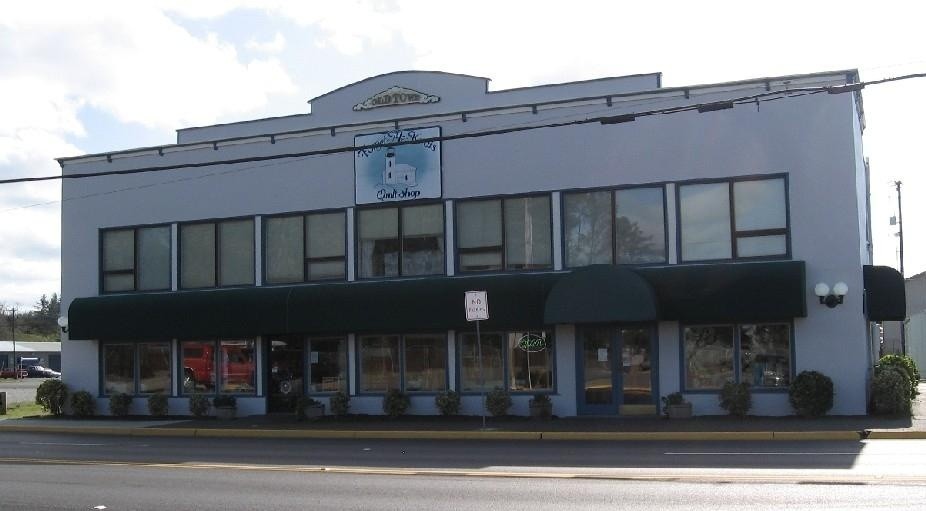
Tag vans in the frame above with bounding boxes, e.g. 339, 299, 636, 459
182, 344, 255, 391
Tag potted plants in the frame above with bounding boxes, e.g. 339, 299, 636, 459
213, 394, 236, 420
529, 393, 552, 421
666, 392, 692, 419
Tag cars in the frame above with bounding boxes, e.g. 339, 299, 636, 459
0, 366, 61, 379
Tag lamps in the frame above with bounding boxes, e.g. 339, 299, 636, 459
56, 316, 68, 334
812, 281, 850, 308
